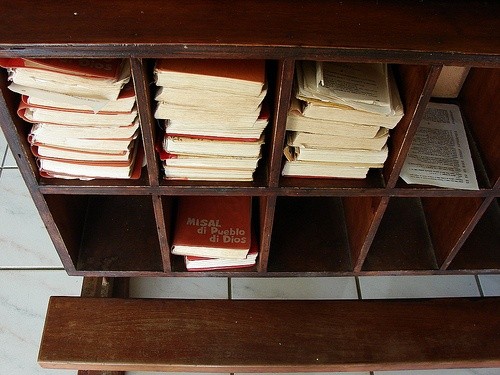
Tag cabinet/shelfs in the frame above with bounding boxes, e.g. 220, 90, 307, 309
1, 0, 500, 276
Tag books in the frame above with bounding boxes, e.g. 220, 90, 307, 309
171, 195, 253, 259
0, 58, 147, 180
153, 60, 269, 181
431, 65, 472, 98
282, 61, 404, 180
185, 236, 259, 271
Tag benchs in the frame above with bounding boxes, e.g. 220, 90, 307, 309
37, 296, 499, 375
